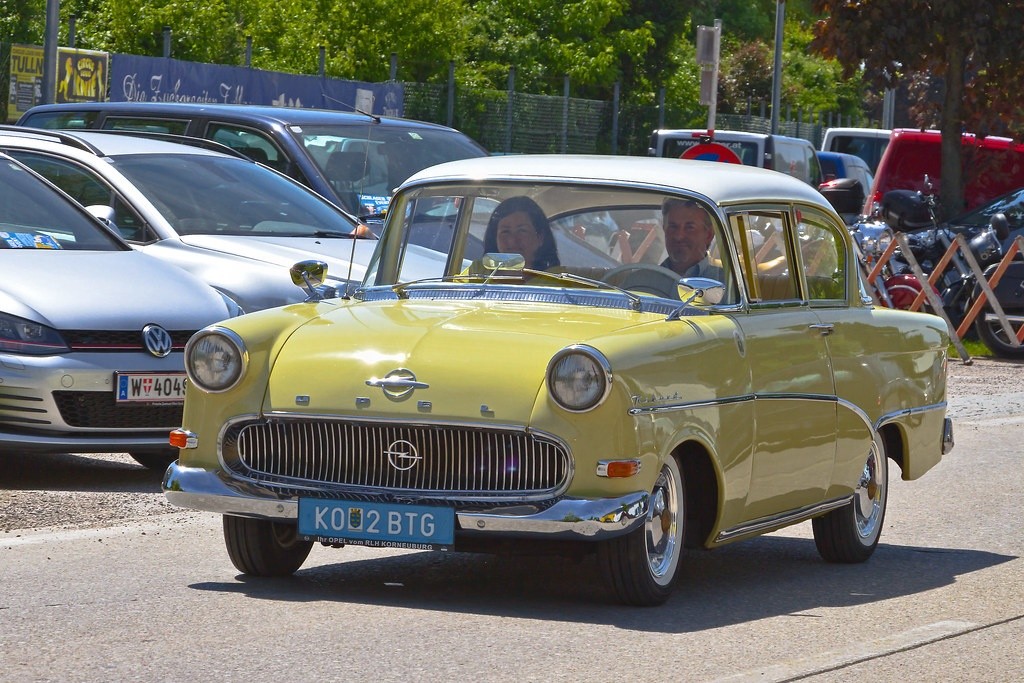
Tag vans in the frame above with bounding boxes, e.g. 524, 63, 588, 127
11, 101, 627, 286
813, 149, 877, 225
649, 125, 824, 191
863, 130, 1024, 231
821, 127, 895, 176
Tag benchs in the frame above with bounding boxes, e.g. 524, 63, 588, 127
567, 264, 844, 301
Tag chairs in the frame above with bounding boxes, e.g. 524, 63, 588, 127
326, 151, 372, 192
232, 147, 269, 165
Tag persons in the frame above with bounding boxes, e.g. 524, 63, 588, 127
452, 195, 627, 287
620, 195, 725, 308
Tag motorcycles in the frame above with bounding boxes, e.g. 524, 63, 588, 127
817, 178, 1024, 358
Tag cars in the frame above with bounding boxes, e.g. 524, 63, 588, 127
160, 152, 955, 605
0, 154, 247, 469
0, 121, 476, 320
